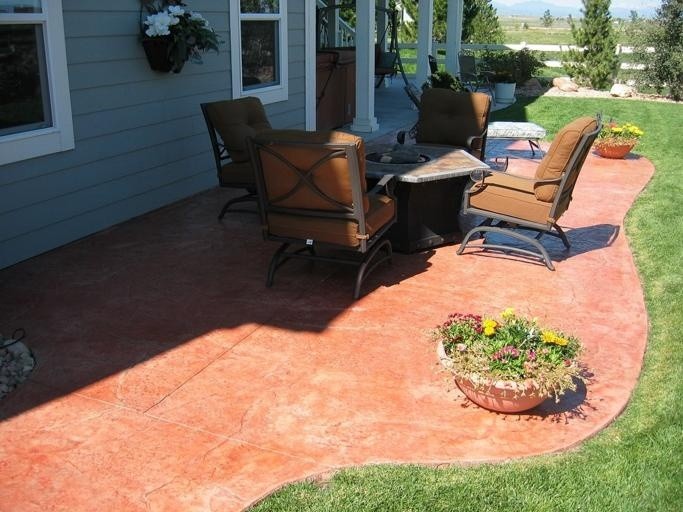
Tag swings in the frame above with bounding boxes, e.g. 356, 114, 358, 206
319, 4, 396, 88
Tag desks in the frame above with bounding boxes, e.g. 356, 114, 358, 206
357, 143, 493, 255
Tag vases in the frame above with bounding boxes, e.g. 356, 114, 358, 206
435, 341, 560, 415
593, 139, 640, 159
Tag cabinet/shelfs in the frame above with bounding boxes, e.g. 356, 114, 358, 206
315, 46, 358, 132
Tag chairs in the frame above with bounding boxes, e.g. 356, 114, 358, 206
453, 114, 605, 273
456, 49, 496, 101
243, 125, 402, 303
407, 86, 496, 192
428, 54, 438, 76
375, 52, 399, 91
199, 96, 306, 221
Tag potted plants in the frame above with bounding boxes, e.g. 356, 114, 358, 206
477, 51, 542, 104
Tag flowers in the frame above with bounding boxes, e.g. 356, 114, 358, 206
427, 308, 598, 402
593, 121, 647, 145
420, 70, 469, 94
137, 0, 224, 76
